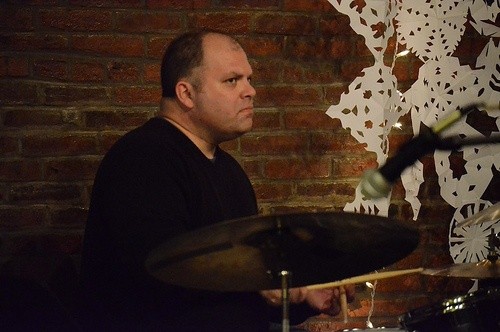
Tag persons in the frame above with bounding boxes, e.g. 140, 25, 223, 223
80, 28, 355, 332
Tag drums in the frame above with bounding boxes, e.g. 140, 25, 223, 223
397, 287, 500, 332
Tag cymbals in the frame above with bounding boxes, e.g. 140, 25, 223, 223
457, 202, 500, 229
144, 211, 422, 293
423, 261, 500, 279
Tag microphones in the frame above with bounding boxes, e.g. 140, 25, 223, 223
360, 102, 478, 199
